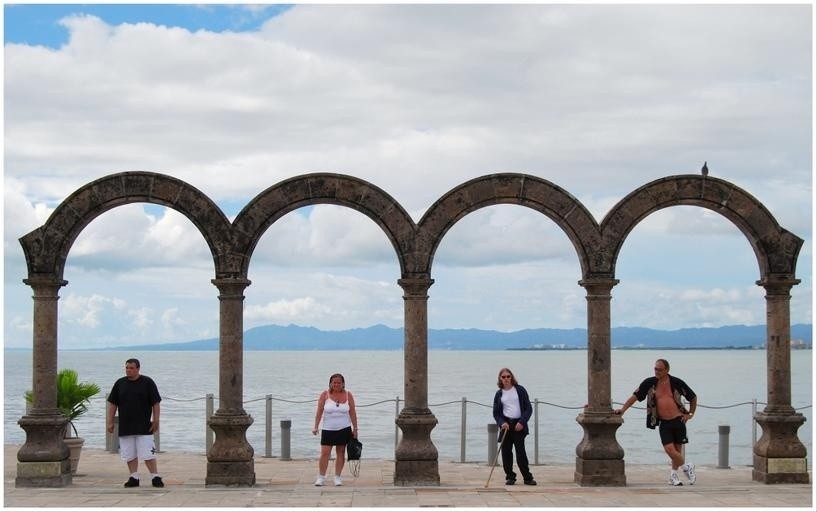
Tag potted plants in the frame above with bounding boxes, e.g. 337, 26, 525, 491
24, 370, 102, 476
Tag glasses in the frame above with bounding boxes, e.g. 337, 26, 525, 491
501, 375, 511, 378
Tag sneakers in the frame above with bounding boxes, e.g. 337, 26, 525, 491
524, 480, 536, 485
124, 477, 139, 487
505, 479, 516, 485
669, 474, 683, 486
684, 465, 696, 485
315, 477, 325, 486
335, 477, 342, 485
152, 476, 163, 487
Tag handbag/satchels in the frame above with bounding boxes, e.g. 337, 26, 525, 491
348, 438, 362, 461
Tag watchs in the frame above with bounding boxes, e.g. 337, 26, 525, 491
687, 412, 693, 417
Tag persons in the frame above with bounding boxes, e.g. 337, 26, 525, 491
491, 367, 538, 485
613, 358, 697, 486
106, 357, 165, 488
310, 373, 360, 486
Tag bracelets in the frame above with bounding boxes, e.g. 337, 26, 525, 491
352, 427, 359, 430
